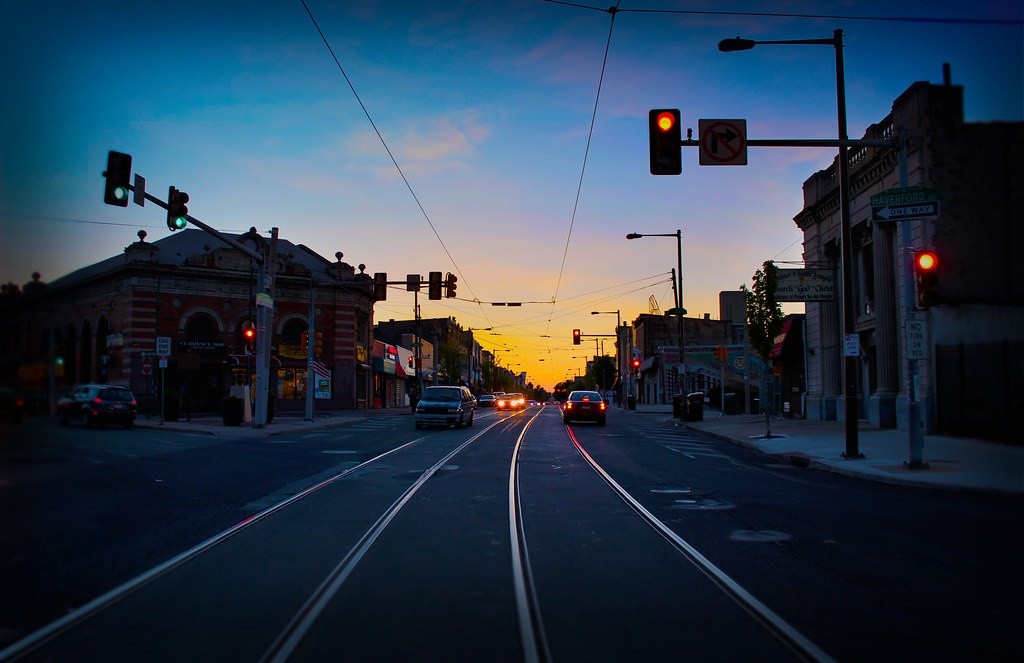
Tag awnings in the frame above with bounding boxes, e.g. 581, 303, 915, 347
769, 318, 803, 359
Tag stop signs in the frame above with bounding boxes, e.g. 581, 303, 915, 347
142, 357, 153, 375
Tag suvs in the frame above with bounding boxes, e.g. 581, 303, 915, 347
56, 384, 138, 427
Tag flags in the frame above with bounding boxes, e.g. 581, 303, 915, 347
313, 356, 330, 379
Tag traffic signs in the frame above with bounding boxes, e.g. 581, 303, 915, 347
140, 350, 156, 357
872, 201, 940, 221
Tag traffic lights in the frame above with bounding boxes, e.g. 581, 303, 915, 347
167, 186, 188, 231
648, 108, 682, 175
104, 150, 132, 207
408, 357, 413, 367
418, 359, 422, 368
245, 328, 255, 344
573, 329, 580, 345
634, 360, 639, 374
914, 252, 940, 309
445, 272, 457, 298
314, 332, 323, 356
713, 346, 721, 360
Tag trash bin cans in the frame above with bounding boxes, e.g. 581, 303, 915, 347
672, 394, 684, 418
687, 391, 704, 422
628, 395, 636, 410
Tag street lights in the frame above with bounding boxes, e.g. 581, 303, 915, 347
568, 368, 580, 382
572, 356, 587, 363
493, 349, 510, 365
566, 373, 576, 380
468, 327, 491, 390
591, 310, 622, 408
580, 338, 598, 356
626, 230, 685, 363
718, 28, 858, 458
507, 364, 520, 370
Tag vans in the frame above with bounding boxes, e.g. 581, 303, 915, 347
414, 386, 474, 429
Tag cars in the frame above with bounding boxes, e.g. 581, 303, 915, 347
0, 388, 24, 424
562, 390, 606, 425
471, 392, 561, 411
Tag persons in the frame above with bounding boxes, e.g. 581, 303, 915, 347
409, 382, 419, 412
